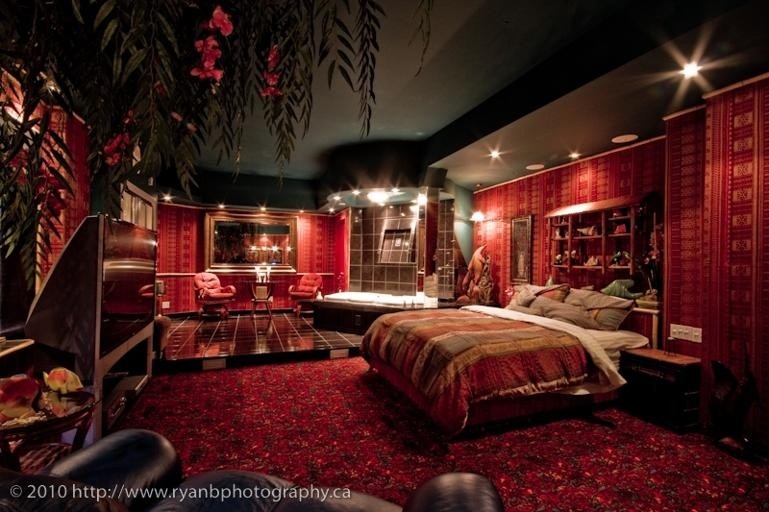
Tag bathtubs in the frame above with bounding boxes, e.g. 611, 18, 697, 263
325, 291, 433, 305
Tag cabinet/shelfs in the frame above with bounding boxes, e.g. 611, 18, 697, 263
543, 194, 642, 274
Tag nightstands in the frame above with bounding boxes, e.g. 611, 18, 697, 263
619, 347, 704, 433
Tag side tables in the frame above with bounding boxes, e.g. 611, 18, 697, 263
0, 379, 100, 478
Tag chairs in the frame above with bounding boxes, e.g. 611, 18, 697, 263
193, 272, 237, 323
288, 273, 325, 319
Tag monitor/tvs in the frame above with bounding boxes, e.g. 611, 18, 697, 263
17, 215, 157, 368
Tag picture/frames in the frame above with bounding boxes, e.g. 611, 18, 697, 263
507, 216, 532, 285
199, 210, 300, 276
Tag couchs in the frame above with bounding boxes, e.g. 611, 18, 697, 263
1, 419, 501, 512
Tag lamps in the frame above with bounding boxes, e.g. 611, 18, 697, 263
468, 211, 491, 224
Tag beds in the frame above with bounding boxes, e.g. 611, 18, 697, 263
359, 284, 662, 436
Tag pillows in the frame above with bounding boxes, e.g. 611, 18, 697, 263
506, 283, 569, 321
563, 287, 634, 333
